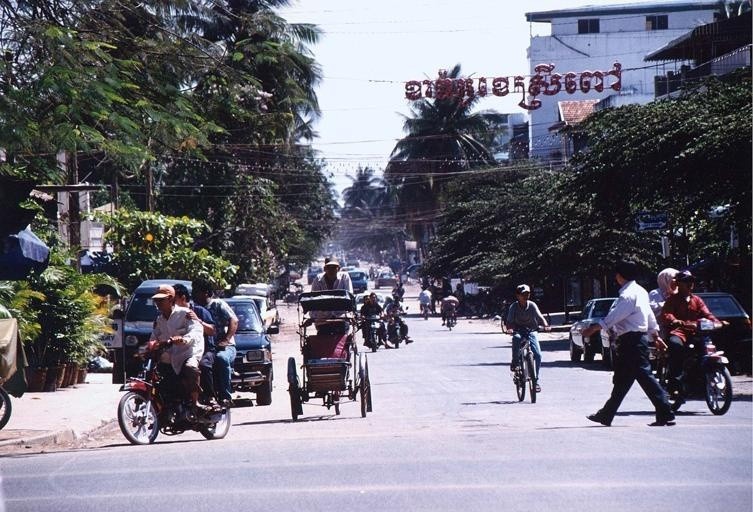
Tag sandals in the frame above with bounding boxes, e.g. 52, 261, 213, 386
536, 383, 540, 392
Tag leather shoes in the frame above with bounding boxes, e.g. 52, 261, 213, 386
649, 419, 676, 426
586, 413, 611, 426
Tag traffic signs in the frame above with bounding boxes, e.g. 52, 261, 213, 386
635, 210, 669, 232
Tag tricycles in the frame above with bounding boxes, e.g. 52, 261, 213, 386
287, 287, 372, 419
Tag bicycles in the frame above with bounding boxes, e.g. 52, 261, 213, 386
502, 324, 547, 403
0, 387, 13, 427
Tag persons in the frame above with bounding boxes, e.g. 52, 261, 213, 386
648, 267, 681, 340
505, 284, 551, 393
312, 250, 470, 348
661, 270, 730, 381
582, 260, 676, 427
138, 278, 239, 418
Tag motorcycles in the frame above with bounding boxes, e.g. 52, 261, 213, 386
455, 290, 513, 320
117, 339, 233, 446
416, 297, 430, 320
655, 318, 733, 415
444, 310, 457, 331
382, 306, 409, 348
358, 312, 384, 352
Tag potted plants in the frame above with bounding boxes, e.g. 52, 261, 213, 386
0, 255, 126, 393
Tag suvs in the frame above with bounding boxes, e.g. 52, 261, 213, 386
112, 279, 194, 384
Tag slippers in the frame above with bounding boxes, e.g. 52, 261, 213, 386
210, 399, 221, 411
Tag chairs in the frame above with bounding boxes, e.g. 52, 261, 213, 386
306, 332, 350, 374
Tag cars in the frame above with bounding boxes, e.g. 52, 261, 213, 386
692, 291, 752, 376
306, 253, 421, 320
229, 266, 301, 337
566, 297, 616, 364
199, 297, 274, 404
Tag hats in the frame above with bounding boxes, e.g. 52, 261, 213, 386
676, 270, 696, 282
515, 284, 531, 294
152, 284, 176, 299
324, 258, 340, 266
